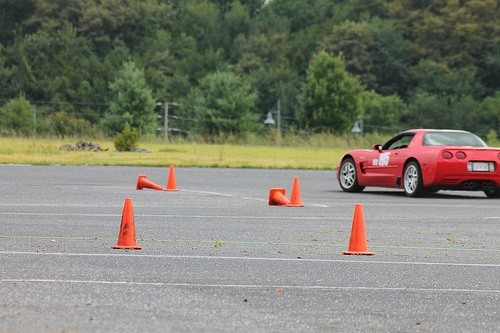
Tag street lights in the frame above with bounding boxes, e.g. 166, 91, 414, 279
264, 98, 281, 132
352, 118, 364, 136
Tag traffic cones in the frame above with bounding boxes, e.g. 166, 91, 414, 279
287, 176, 304, 207
342, 204, 376, 255
163, 165, 180, 191
111, 198, 142, 250
269, 189, 290, 206
136, 175, 163, 190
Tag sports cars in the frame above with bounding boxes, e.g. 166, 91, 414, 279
336, 128, 500, 199
